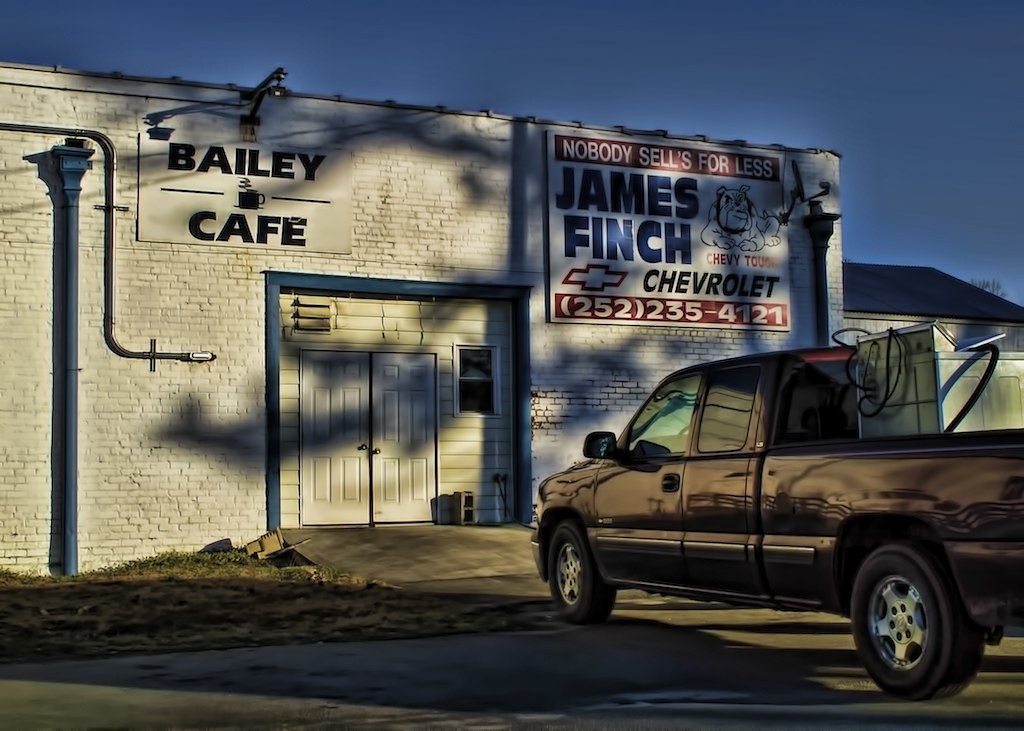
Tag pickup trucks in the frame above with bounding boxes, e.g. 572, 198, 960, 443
529, 344, 1024, 703
